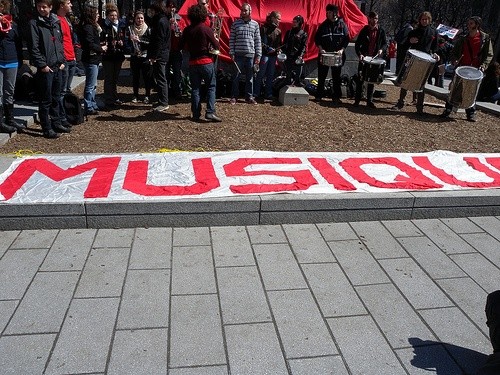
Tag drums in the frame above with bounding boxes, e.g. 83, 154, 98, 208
394, 49, 437, 93
359, 55, 387, 85
320, 52, 342, 67
447, 66, 484, 110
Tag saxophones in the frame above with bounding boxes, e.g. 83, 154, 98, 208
126, 25, 144, 59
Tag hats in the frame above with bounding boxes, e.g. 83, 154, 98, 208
473, 15, 483, 27
325, 4, 338, 11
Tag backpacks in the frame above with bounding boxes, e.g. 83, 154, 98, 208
327, 79, 334, 97
349, 74, 363, 94
63, 92, 84, 124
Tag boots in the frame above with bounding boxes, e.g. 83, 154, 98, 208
0, 106, 18, 133
3, 104, 26, 130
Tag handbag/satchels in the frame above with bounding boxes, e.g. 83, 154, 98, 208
305, 82, 326, 97
340, 74, 350, 98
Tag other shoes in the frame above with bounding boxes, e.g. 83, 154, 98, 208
192, 117, 199, 122
143, 96, 150, 103
229, 98, 237, 105
62, 120, 72, 128
467, 118, 476, 122
99, 106, 111, 111
313, 97, 322, 102
440, 109, 451, 118
152, 104, 170, 112
205, 113, 222, 122
54, 125, 69, 133
332, 98, 341, 104
245, 95, 257, 105
43, 128, 60, 138
131, 96, 138, 103
87, 110, 99, 115
111, 103, 119, 109
390, 101, 405, 110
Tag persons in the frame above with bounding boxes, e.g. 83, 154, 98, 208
99, 4, 125, 104
314, 4, 349, 102
254, 11, 281, 98
354, 11, 387, 108
81, 5, 101, 114
282, 15, 307, 84
180, 5, 222, 122
391, 11, 440, 115
394, 18, 418, 74
229, 3, 262, 105
428, 35, 454, 88
54, 0, 76, 127
0, 0, 26, 133
146, 1, 170, 110
162, 0, 186, 102
128, 11, 152, 103
27, 0, 70, 138
198, 0, 220, 74
443, 16, 493, 122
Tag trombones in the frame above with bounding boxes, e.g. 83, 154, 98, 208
209, 10, 224, 56
